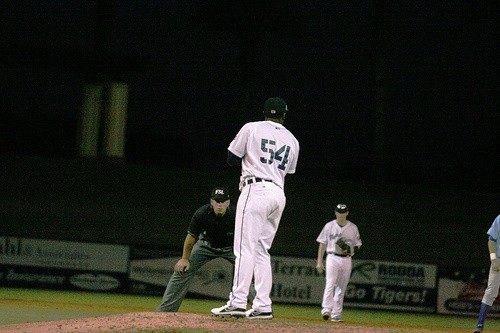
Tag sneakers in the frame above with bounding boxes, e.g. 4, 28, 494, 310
211, 304, 247, 321
241, 309, 274, 320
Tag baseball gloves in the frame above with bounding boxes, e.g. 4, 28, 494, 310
336, 238, 354, 255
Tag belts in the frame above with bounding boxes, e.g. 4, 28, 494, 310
330, 253, 351, 257
242, 178, 273, 187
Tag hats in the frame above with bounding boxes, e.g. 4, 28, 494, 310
262, 97, 287, 119
335, 203, 349, 213
211, 187, 230, 202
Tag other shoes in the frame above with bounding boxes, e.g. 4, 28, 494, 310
331, 317, 344, 322
473, 328, 484, 333
322, 312, 330, 320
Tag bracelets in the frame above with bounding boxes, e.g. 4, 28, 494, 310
490, 252, 497, 260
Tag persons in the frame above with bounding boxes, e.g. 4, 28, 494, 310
156, 187, 256, 313
315, 204, 363, 322
210, 97, 299, 319
473, 215, 500, 333
